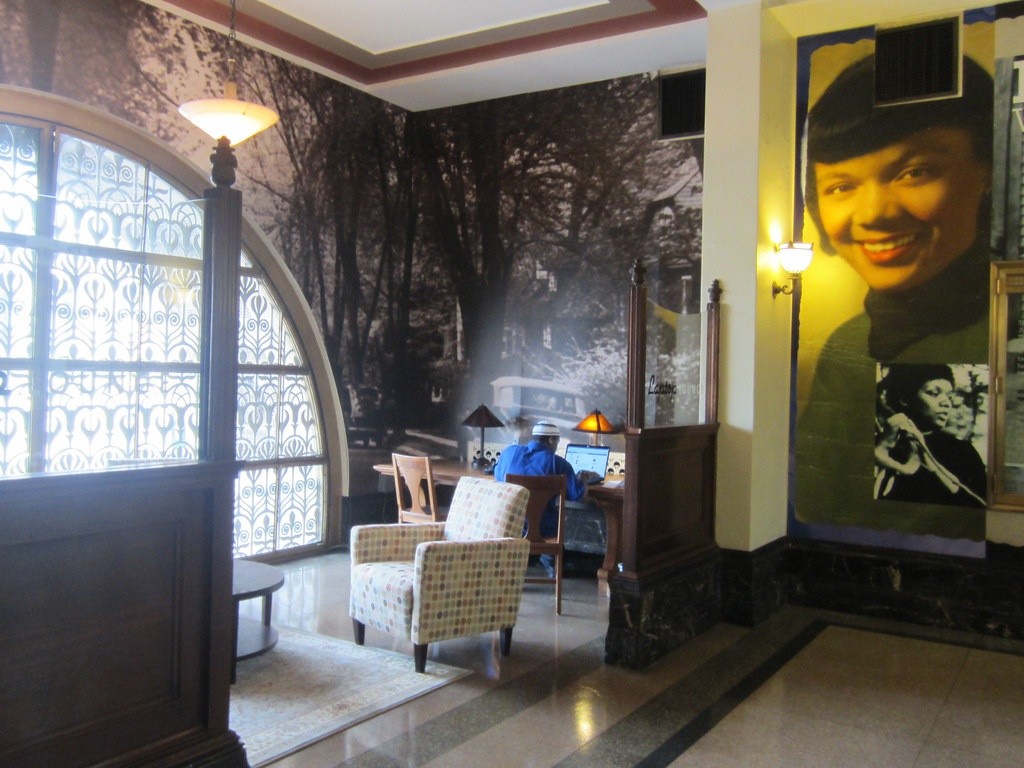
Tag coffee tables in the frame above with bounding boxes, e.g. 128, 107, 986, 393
230, 559, 286, 685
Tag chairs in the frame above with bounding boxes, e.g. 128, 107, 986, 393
389, 453, 447, 525
505, 474, 567, 616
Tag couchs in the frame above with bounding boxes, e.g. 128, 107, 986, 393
349, 476, 531, 674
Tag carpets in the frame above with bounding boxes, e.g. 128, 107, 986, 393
228, 626, 474, 768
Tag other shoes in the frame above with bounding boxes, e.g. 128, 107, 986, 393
536, 557, 554, 579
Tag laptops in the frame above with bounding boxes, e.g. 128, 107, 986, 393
565, 444, 610, 484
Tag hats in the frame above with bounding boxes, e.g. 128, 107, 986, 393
532, 420, 561, 436
885, 363, 956, 408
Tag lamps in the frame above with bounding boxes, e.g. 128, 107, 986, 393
179, 0, 281, 147
772, 247, 813, 298
571, 408, 620, 446
460, 403, 506, 468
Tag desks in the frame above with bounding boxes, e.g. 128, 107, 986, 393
372, 458, 625, 598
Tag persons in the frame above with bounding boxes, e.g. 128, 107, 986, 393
604, 461, 626, 476
494, 419, 592, 579
471, 450, 501, 476
797, 47, 997, 541
